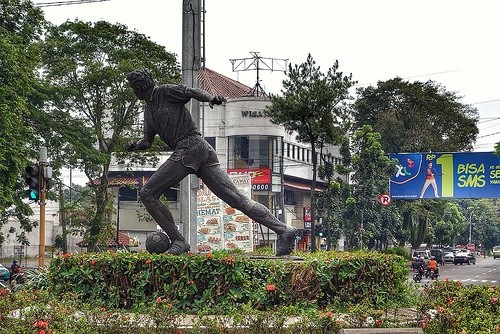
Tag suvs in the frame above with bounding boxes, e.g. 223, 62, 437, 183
407, 243, 476, 266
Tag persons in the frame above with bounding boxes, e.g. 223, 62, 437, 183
10, 260, 19, 271
126, 68, 298, 255
428, 256, 438, 270
412, 252, 430, 276
420, 162, 443, 198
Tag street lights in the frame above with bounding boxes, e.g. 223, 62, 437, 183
469, 206, 480, 244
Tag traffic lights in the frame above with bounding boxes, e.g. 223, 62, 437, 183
25, 164, 42, 200
316, 230, 334, 237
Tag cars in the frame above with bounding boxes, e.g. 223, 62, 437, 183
0, 263, 11, 281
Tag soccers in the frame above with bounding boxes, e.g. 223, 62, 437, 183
146, 232, 170, 254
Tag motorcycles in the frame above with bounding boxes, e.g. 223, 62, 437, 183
412, 266, 440, 283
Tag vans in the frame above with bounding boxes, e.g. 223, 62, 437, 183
493, 246, 500, 259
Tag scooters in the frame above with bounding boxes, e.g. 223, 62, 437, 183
9, 266, 26, 284
